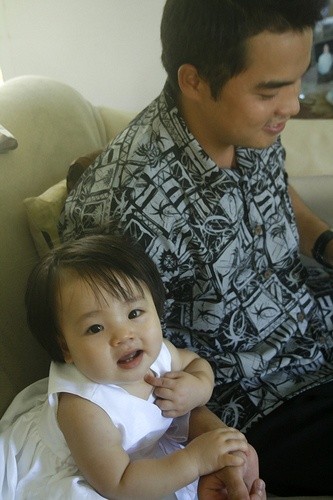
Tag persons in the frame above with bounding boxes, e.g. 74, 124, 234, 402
0, 234, 251, 500
59, 0, 333, 500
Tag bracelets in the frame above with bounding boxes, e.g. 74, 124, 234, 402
310, 230, 333, 270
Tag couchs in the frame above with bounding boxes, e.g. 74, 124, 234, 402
1, 75, 134, 418
